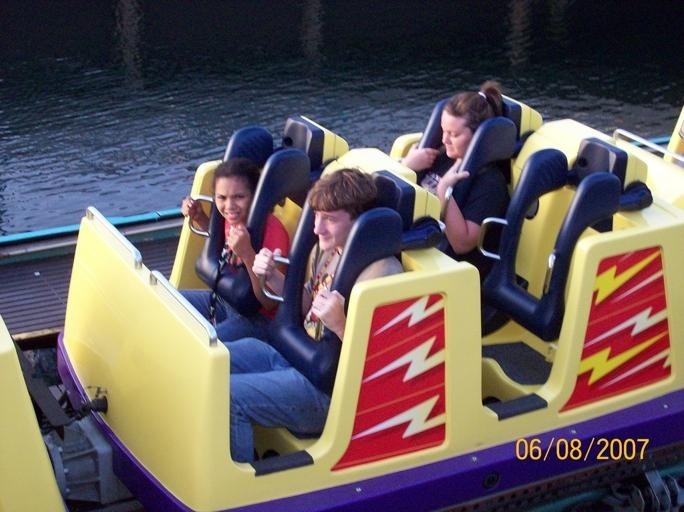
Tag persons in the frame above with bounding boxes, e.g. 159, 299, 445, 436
221, 168, 404, 462
175, 158, 291, 344
399, 79, 508, 286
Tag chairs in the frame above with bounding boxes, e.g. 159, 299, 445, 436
196, 117, 324, 321
257, 170, 424, 455
393, 91, 527, 260
482, 137, 627, 401
